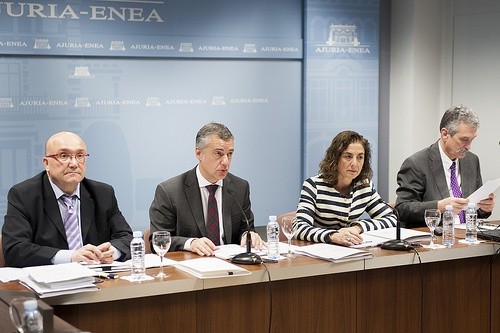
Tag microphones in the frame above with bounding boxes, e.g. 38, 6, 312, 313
227, 187, 262, 265
377, 198, 411, 250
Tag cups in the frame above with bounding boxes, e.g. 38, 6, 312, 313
10, 298, 38, 333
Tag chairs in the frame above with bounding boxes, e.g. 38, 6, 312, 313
278, 212, 296, 240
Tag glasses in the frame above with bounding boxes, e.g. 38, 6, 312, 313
46, 152, 90, 163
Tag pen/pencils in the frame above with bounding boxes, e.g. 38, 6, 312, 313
93, 275, 110, 280
361, 240, 372, 244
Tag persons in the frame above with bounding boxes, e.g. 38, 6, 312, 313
2, 132, 134, 268
393, 106, 496, 229
292, 130, 397, 246
148, 123, 267, 257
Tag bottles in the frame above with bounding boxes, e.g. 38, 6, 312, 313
465, 203, 478, 242
20, 300, 43, 333
266, 216, 281, 258
443, 205, 455, 246
129, 231, 146, 279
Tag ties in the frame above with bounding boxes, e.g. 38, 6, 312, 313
60, 195, 83, 250
451, 162, 465, 223
205, 185, 220, 246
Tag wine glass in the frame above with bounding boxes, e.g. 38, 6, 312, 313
424, 209, 441, 247
281, 217, 298, 259
152, 231, 172, 278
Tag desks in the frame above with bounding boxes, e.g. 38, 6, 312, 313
0, 220, 500, 333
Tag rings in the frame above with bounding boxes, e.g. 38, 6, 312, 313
347, 237, 350, 241
